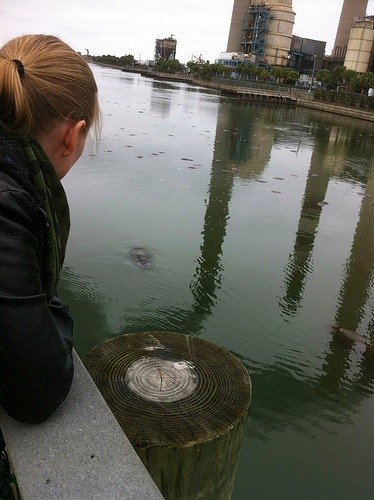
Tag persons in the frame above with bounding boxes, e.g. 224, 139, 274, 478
0, 32, 102, 500
